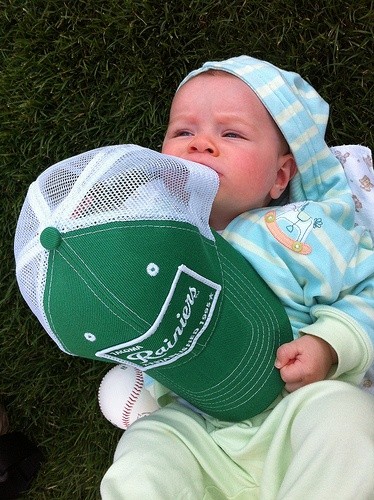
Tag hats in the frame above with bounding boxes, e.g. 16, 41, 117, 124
14, 144, 294, 421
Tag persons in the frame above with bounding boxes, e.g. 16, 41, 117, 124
100, 55, 374, 500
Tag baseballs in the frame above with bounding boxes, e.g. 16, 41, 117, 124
98, 364, 160, 431
